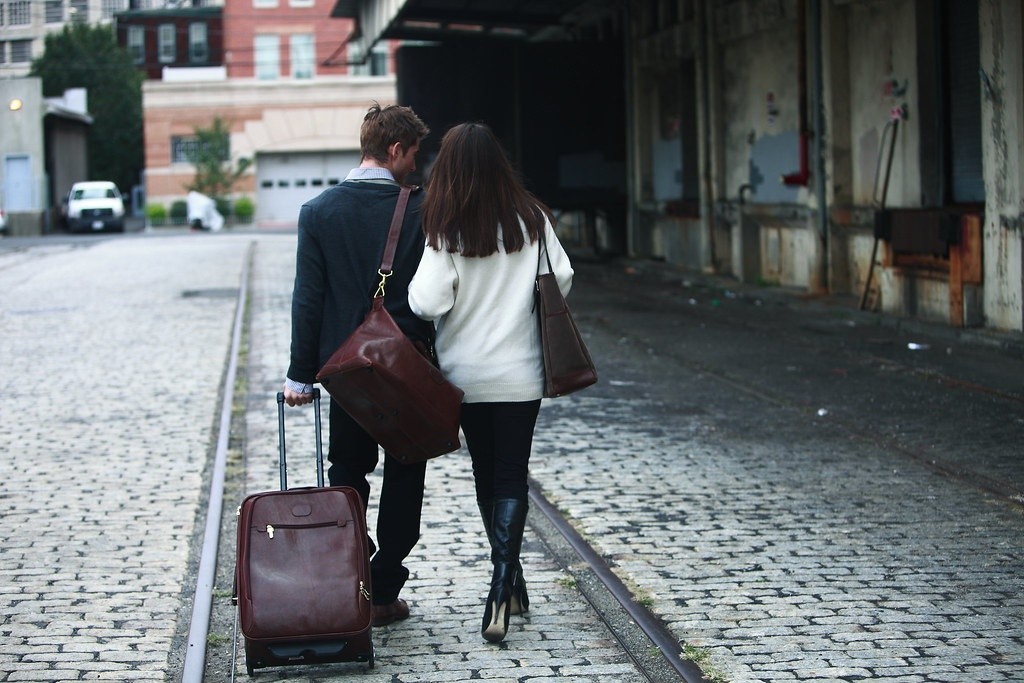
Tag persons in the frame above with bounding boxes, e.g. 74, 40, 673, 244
407, 123, 574, 641
283, 105, 442, 625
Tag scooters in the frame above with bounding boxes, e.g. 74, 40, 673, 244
189, 191, 217, 233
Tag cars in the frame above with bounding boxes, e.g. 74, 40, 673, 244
61, 181, 130, 235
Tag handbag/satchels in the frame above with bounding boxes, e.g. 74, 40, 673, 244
531, 228, 597, 397
316, 296, 465, 463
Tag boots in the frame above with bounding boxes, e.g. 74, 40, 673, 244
478, 498, 529, 615
481, 499, 530, 642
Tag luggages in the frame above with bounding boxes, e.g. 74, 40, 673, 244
232, 388, 376, 676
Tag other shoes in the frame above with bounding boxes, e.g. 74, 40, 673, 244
373, 597, 408, 627
